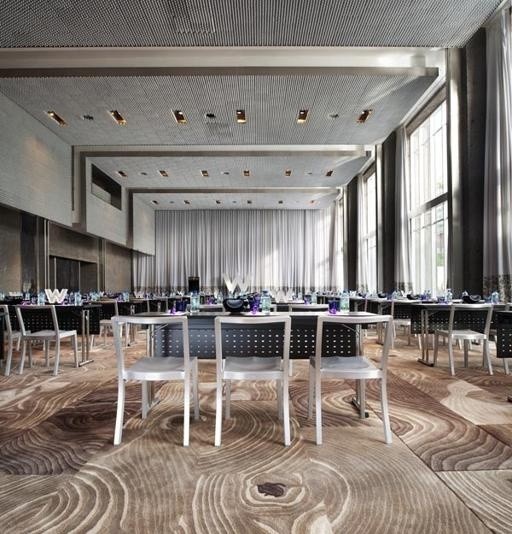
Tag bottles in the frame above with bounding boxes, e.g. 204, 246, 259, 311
446, 289, 452, 303
69, 288, 82, 306
340, 290, 350, 315
310, 290, 318, 306
260, 290, 271, 316
422, 290, 431, 300
24, 289, 30, 300
492, 289, 500, 304
462, 289, 468, 297
190, 290, 200, 315
38, 289, 45, 306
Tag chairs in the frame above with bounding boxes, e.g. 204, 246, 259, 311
0, 295, 511, 446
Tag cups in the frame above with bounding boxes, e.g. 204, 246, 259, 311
328, 300, 337, 315
249, 297, 260, 315
